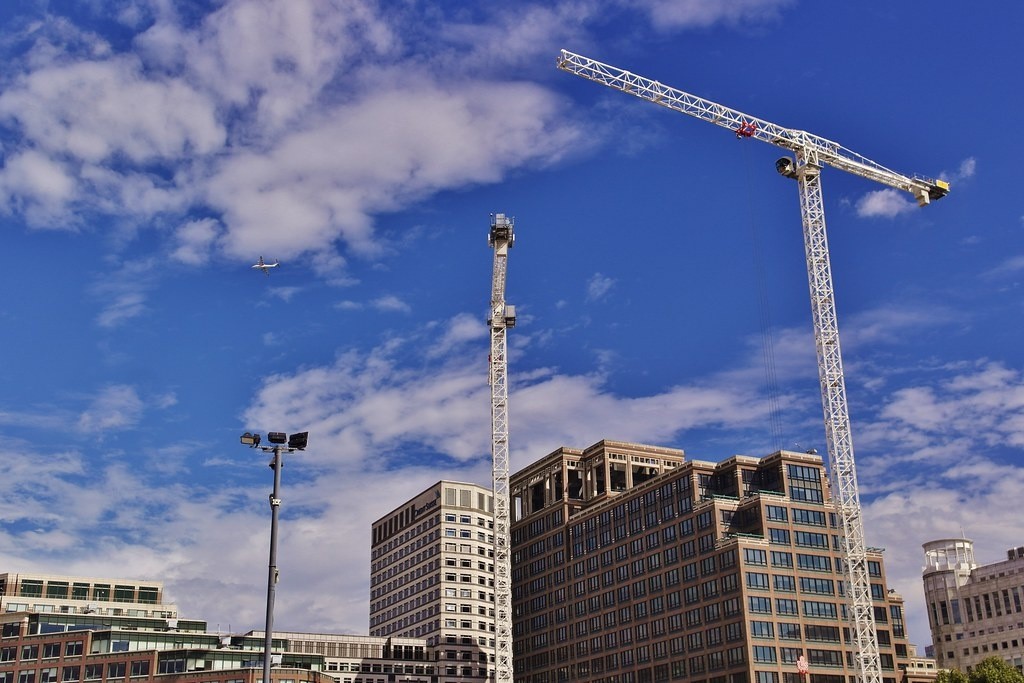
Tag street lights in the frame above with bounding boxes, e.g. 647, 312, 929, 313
239, 432, 308, 683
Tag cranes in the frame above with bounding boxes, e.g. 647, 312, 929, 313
551, 44, 954, 683
481, 209, 519, 683
